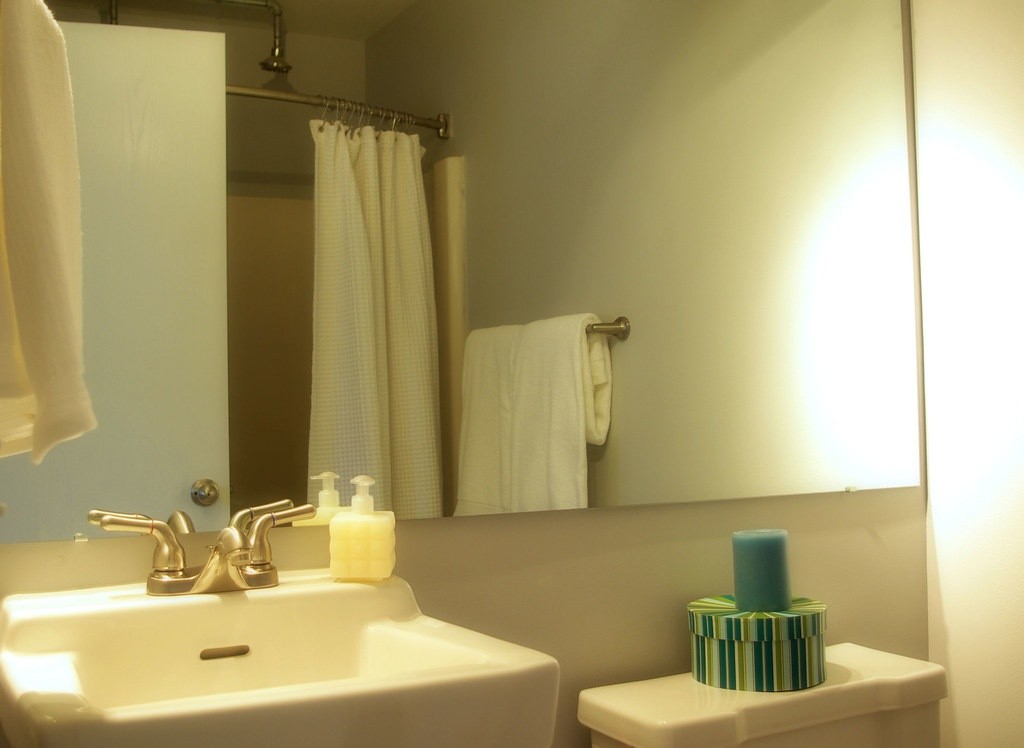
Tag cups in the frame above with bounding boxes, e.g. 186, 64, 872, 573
732, 528, 790, 612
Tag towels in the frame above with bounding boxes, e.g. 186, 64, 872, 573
447, 313, 612, 512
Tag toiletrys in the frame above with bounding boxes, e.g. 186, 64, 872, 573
325, 472, 399, 582
292, 474, 349, 531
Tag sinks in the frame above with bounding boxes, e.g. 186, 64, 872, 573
0, 570, 561, 748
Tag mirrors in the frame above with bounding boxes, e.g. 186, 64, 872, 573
0, 0, 920, 545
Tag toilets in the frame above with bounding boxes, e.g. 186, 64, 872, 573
576, 639, 950, 748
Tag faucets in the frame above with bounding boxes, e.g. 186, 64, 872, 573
89, 504, 318, 592
84, 498, 297, 538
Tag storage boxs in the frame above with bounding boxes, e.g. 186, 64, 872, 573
684, 594, 828, 693
